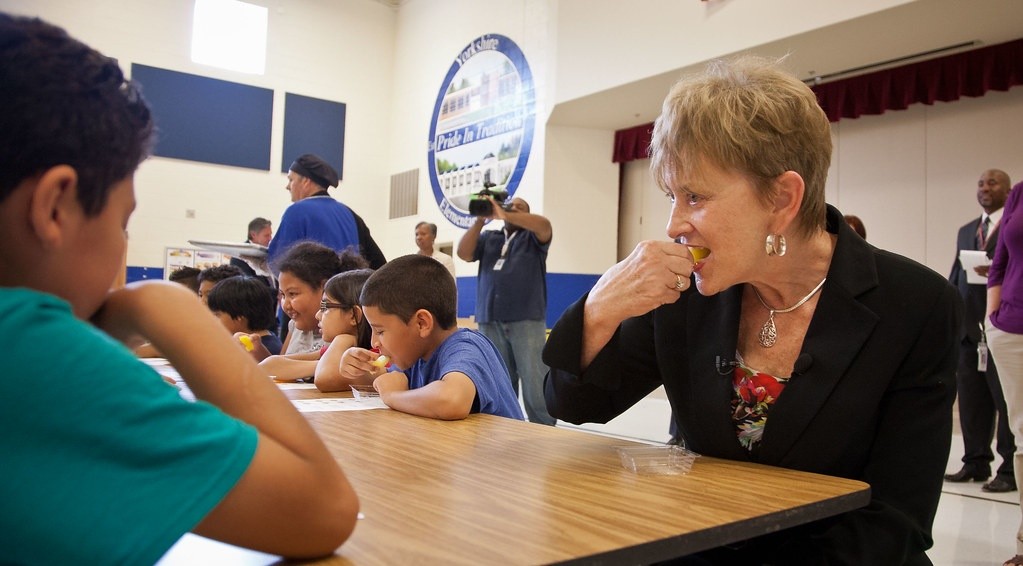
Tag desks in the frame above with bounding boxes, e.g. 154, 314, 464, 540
152, 377, 872, 566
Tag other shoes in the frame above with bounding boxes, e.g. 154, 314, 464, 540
1004, 555, 1023, 566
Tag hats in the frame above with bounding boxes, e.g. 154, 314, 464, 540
289, 155, 339, 189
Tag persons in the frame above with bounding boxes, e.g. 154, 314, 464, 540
845, 215, 866, 240
542, 62, 967, 566
457, 195, 558, 425
135, 242, 391, 394
415, 222, 455, 276
984, 180, 1023, 566
0, 11, 359, 566
340, 254, 525, 420
944, 168, 1017, 491
240, 155, 359, 341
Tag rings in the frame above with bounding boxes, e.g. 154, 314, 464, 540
672, 274, 682, 290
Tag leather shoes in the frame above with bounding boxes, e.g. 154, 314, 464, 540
983, 476, 1017, 493
944, 463, 989, 482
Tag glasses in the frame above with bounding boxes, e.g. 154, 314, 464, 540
320, 300, 352, 312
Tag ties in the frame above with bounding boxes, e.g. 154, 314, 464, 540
982, 216, 991, 241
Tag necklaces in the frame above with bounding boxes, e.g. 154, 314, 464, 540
752, 278, 826, 348
